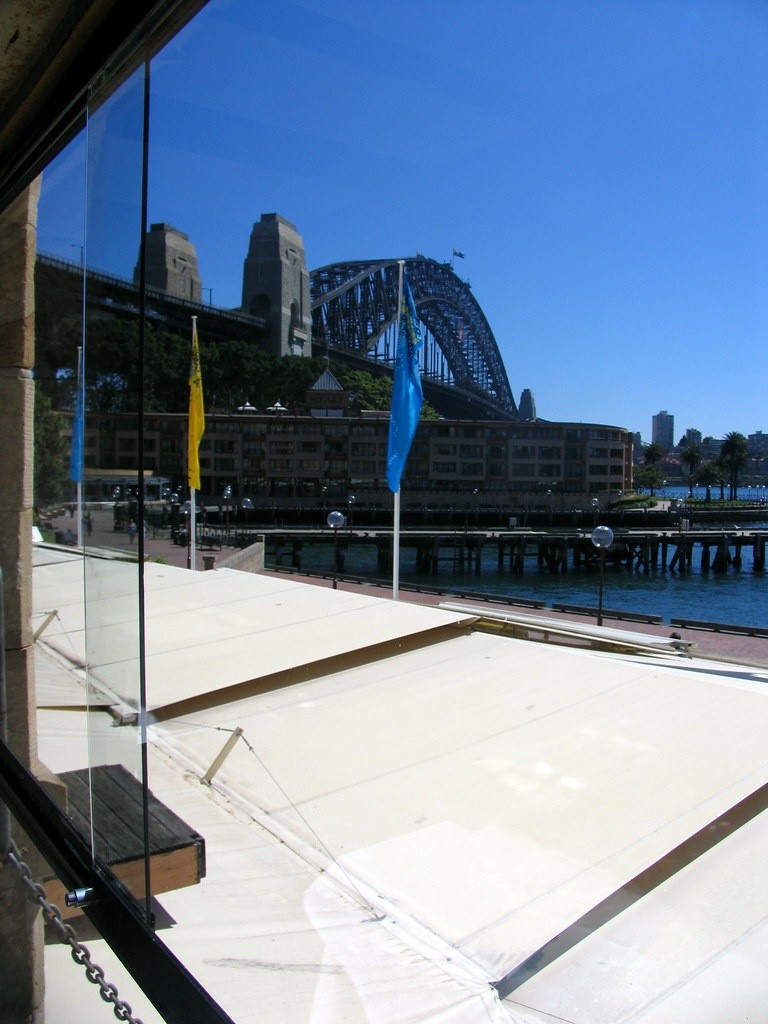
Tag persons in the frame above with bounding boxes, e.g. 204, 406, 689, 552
668, 632, 691, 659
33, 504, 190, 547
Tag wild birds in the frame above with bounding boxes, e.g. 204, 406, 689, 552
734, 525, 741, 529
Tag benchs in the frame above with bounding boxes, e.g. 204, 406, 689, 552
60, 531, 76, 546
198, 536, 223, 552
43, 522, 60, 532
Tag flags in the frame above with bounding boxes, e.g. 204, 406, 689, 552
67, 351, 85, 483
187, 323, 206, 490
385, 266, 423, 492
453, 250, 465, 258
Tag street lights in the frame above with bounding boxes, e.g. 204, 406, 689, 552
590, 525, 614, 628
591, 497, 600, 530
112, 485, 139, 506
695, 483, 766, 501
323, 486, 328, 512
163, 484, 191, 528
662, 480, 666, 501
472, 488, 478, 510
223, 485, 253, 543
327, 511, 344, 589
546, 488, 553, 511
347, 495, 356, 534
